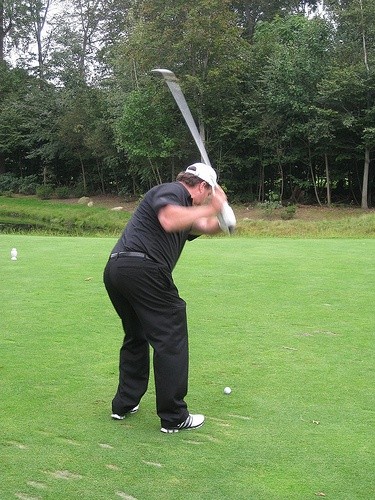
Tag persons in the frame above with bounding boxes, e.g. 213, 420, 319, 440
102, 163, 228, 434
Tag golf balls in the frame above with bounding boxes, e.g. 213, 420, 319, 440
224, 387, 231, 394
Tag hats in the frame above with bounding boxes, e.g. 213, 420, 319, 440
185, 162, 217, 197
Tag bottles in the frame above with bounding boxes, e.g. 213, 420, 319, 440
11, 248, 17, 261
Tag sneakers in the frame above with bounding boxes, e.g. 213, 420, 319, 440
110, 404, 140, 419
161, 414, 205, 433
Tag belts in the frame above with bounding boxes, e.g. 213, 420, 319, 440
110, 252, 153, 260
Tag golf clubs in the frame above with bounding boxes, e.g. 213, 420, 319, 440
151, 68, 234, 234
